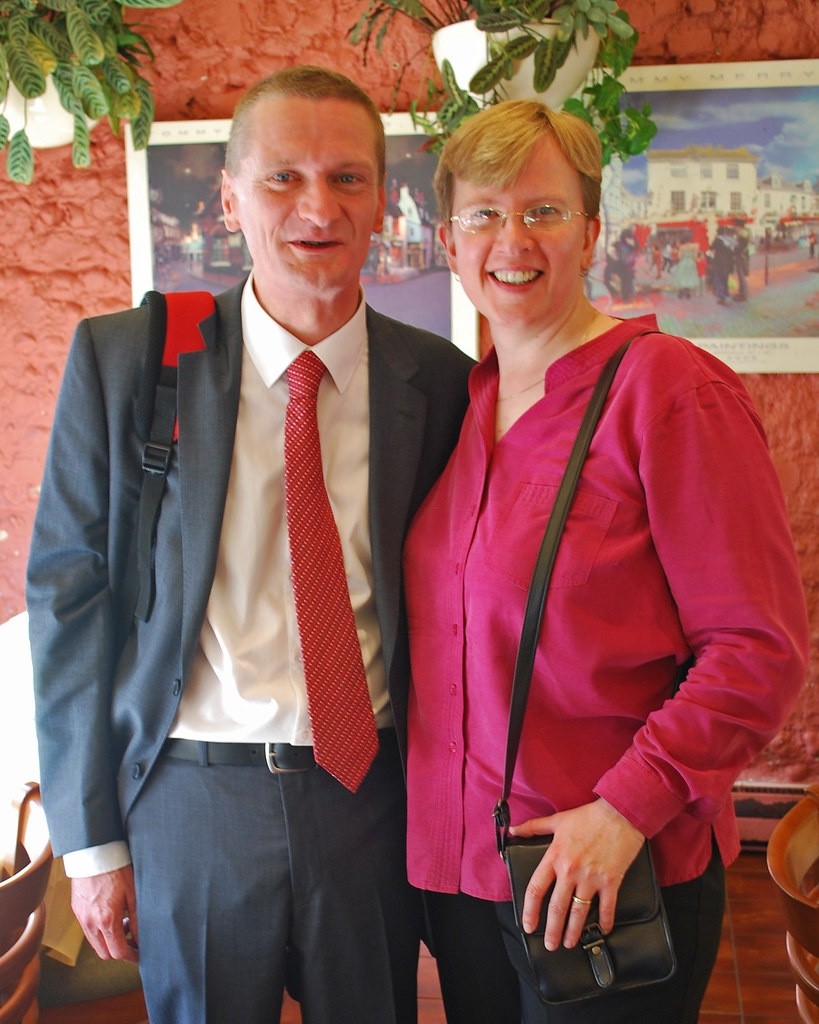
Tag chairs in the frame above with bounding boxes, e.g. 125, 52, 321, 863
764, 785, 819, 1024
0, 780, 58, 1024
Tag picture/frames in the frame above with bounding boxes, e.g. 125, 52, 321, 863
115, 111, 484, 369
562, 57, 819, 374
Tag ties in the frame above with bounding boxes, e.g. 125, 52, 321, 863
283, 350, 379, 795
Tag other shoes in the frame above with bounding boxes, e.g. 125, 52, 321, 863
734, 296, 747, 302
716, 300, 726, 305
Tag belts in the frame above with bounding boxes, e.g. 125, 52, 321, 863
161, 728, 398, 770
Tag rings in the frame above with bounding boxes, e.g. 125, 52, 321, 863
572, 896, 592, 907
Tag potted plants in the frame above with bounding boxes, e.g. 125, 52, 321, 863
344, 0, 661, 177
0, 0, 186, 188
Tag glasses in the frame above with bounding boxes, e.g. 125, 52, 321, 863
450, 203, 591, 234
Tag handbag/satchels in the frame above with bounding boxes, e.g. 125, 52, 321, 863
501, 833, 677, 1008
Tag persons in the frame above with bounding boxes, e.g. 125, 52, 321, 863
25, 66, 485, 1023
602, 213, 759, 305
398, 100, 808, 1024
